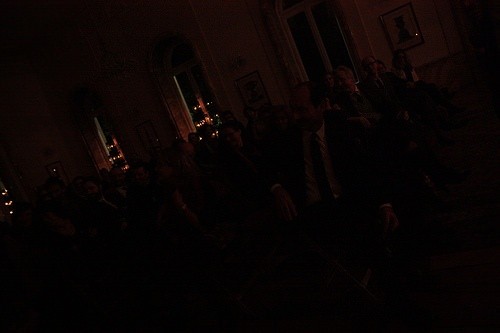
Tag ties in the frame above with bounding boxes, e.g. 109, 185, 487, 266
354, 92, 364, 106
306, 133, 337, 209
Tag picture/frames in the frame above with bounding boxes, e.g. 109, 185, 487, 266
380, 2, 425, 55
235, 71, 270, 110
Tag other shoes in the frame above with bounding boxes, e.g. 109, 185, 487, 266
447, 105, 465, 118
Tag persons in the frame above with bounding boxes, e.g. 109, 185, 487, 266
0, 50, 464, 309
